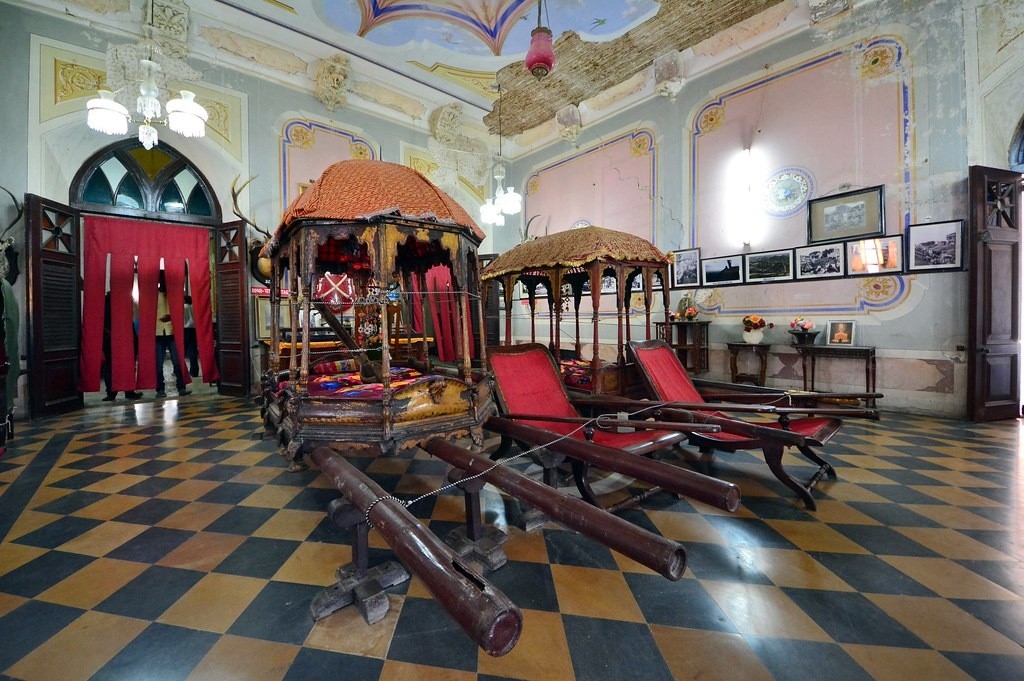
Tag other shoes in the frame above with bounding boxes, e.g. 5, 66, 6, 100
179, 389, 192, 396
125, 391, 143, 399
103, 393, 118, 401
156, 390, 166, 397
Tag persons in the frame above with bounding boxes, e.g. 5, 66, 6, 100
101, 290, 144, 401
171, 295, 199, 377
156, 270, 193, 398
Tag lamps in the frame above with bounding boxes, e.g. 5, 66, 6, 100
84, 1, 210, 153
479, 85, 523, 226
526, 1, 556, 85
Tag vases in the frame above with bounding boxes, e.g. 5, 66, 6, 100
742, 315, 776, 344
787, 318, 820, 346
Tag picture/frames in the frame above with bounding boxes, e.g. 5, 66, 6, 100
907, 217, 966, 274
805, 184, 887, 249
791, 242, 845, 281
826, 319, 857, 347
669, 247, 701, 290
700, 254, 745, 289
516, 262, 670, 299
743, 248, 793, 285
841, 233, 904, 278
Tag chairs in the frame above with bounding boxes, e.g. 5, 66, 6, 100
482, 342, 692, 521
629, 338, 847, 511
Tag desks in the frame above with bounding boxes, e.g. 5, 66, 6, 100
797, 342, 880, 413
726, 341, 769, 386
652, 319, 712, 376
